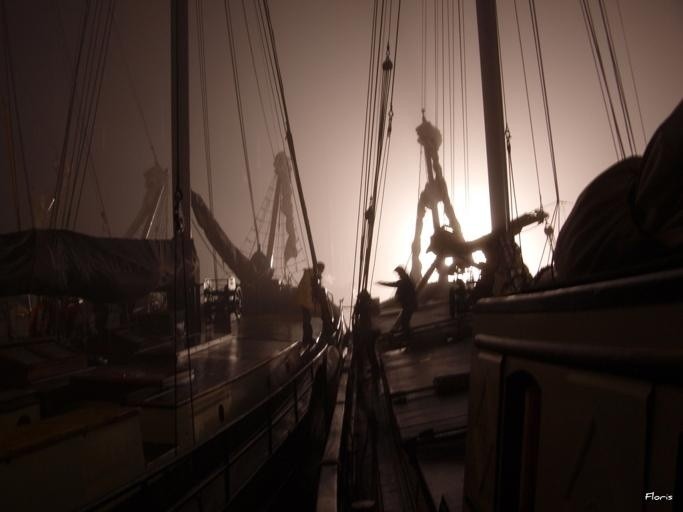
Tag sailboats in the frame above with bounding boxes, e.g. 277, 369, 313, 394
0, 0, 341, 512
342, 0, 683, 511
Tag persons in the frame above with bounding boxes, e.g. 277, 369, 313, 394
376, 266, 417, 331
290, 263, 325, 343
